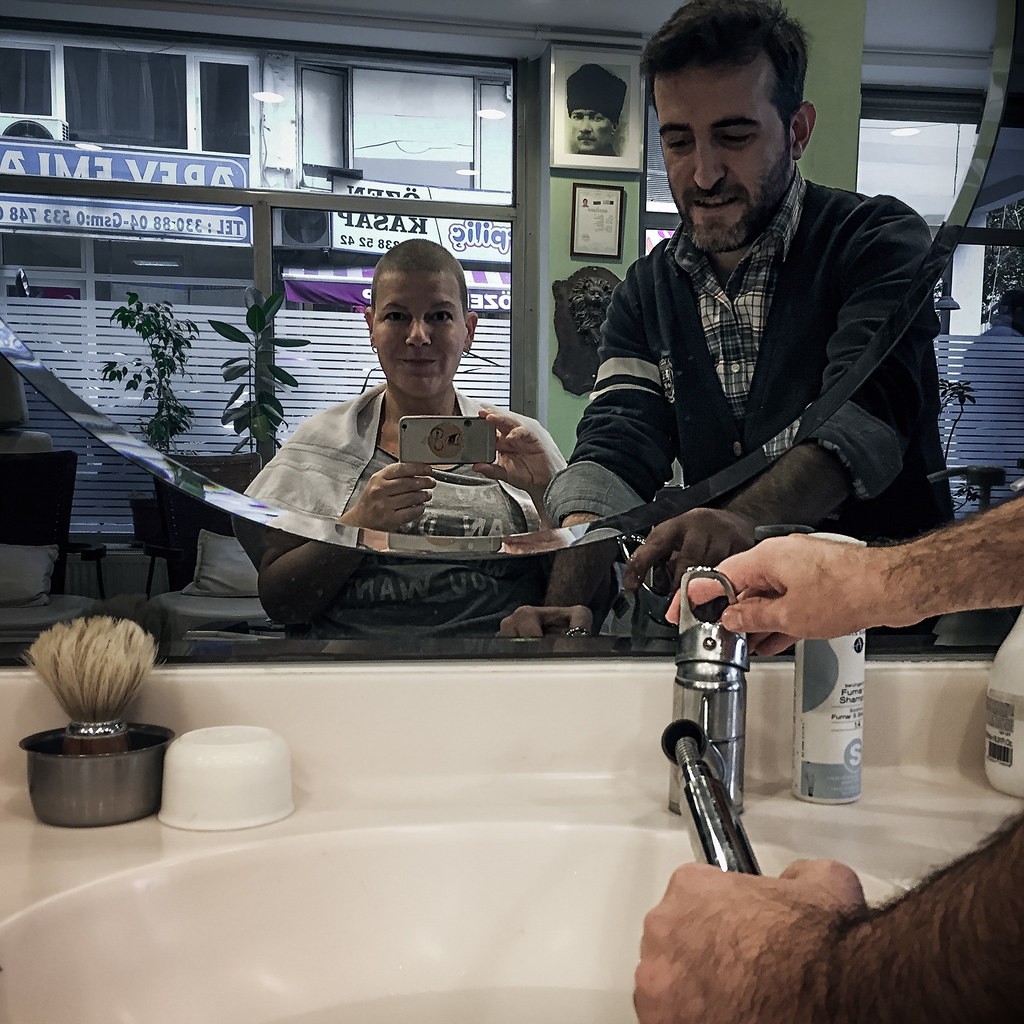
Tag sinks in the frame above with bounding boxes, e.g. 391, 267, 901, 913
2, 823, 895, 1024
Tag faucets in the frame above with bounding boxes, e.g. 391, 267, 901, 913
546, 531, 678, 653
668, 564, 768, 877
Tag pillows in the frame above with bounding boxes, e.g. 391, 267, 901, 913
0, 545, 59, 609
183, 530, 259, 599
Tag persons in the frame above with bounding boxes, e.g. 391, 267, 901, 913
582, 199, 589, 207
564, 63, 626, 157
498, 0, 954, 657
633, 498, 1024, 1024
946, 288, 1024, 522
231, 240, 572, 652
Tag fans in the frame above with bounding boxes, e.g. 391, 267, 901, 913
273, 208, 331, 250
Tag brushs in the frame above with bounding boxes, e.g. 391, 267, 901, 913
16, 615, 170, 756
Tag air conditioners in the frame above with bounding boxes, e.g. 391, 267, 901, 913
0, 113, 69, 142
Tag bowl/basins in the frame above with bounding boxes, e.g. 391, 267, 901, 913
18, 722, 177, 828
155, 725, 298, 830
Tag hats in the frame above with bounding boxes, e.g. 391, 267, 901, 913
565, 63, 627, 122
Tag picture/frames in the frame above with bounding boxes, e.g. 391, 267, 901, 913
570, 182, 623, 260
551, 45, 642, 174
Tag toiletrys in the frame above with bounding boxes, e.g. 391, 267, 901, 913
983, 477, 1024, 798
923, 463, 1009, 646
794, 531, 868, 804
753, 523, 809, 545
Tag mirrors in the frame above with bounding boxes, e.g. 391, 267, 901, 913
0, 0, 1018, 562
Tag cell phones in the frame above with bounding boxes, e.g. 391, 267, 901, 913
398, 416, 497, 465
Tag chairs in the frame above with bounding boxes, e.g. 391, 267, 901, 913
0, 355, 288, 663
